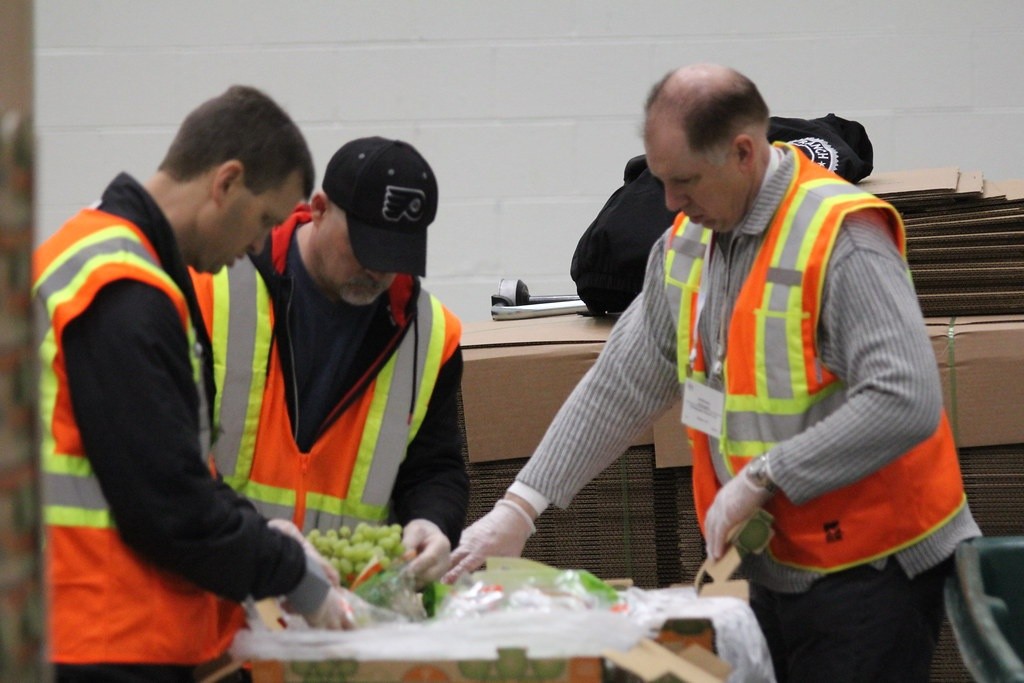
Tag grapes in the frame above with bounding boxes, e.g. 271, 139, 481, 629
304, 522, 410, 593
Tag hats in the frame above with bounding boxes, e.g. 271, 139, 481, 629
321, 135, 439, 277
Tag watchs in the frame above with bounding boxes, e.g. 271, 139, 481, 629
747, 453, 782, 495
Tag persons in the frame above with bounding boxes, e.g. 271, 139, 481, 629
29, 84, 358, 683
185, 137, 468, 591
440, 62, 984, 683
